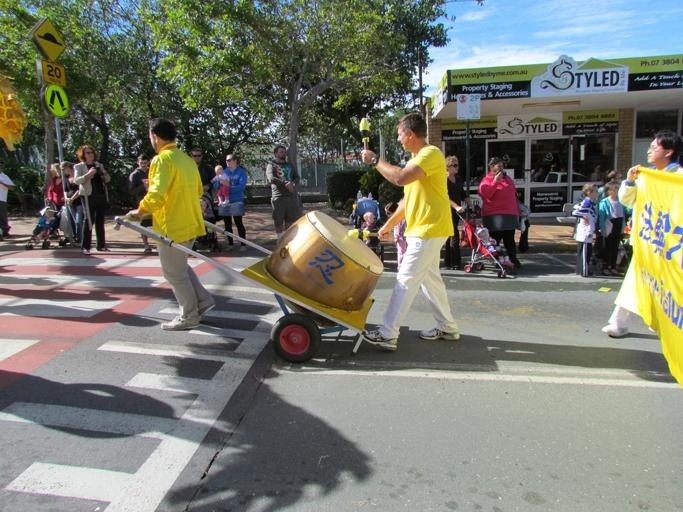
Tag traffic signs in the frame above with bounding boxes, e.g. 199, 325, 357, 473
37, 60, 67, 86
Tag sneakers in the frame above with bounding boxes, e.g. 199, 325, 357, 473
419, 326, 460, 340
602, 325, 629, 337
199, 303, 215, 315
359, 330, 398, 352
161, 315, 200, 330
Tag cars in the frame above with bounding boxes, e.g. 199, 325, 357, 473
533, 169, 590, 182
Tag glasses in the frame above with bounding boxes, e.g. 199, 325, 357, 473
193, 155, 201, 157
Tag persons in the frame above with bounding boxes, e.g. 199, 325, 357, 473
477, 156, 524, 269
265, 144, 306, 243
569, 182, 600, 278
596, 169, 627, 204
355, 214, 376, 248
442, 156, 470, 269
357, 113, 459, 352
598, 180, 628, 276
601, 129, 683, 339
384, 201, 411, 275
0, 160, 15, 241
26, 144, 247, 255
125, 118, 217, 334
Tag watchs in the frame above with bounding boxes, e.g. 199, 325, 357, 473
367, 154, 381, 169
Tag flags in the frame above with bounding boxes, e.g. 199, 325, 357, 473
633, 168, 683, 388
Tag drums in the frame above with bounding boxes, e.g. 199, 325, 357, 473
266, 211, 384, 327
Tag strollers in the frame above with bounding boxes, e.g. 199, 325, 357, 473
25, 198, 70, 251
191, 194, 226, 254
343, 198, 386, 265
454, 206, 516, 279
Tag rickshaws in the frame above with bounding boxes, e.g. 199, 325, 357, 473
108, 195, 376, 361
